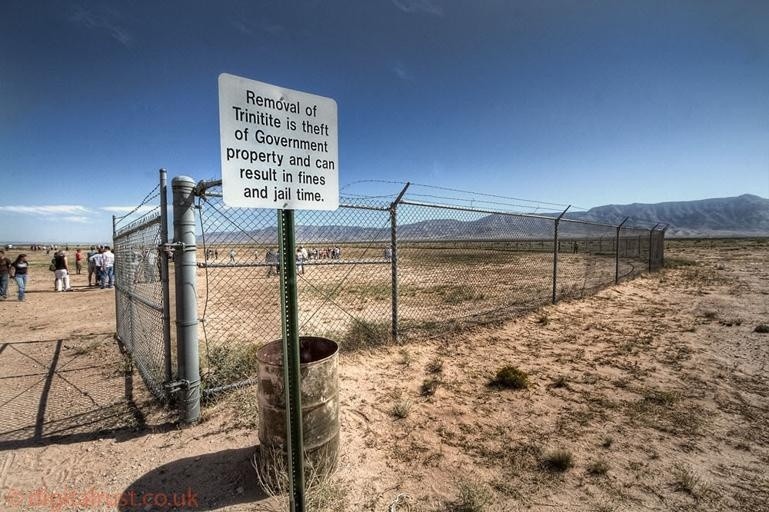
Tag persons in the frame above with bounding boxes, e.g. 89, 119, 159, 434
0, 244, 162, 301
205, 245, 341, 279
388, 249, 393, 262
384, 248, 387, 261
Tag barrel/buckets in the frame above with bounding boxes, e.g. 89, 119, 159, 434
257, 336, 340, 492
